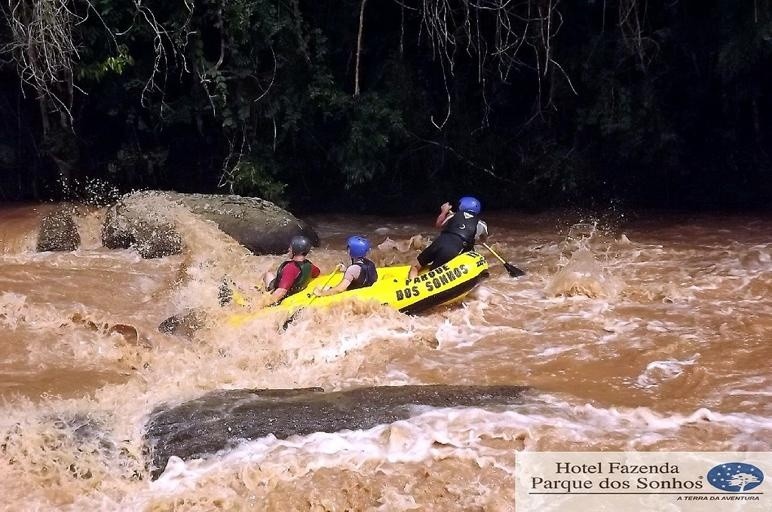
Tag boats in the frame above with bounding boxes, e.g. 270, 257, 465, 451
220, 251, 490, 330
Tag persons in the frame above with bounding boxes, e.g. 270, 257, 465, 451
408, 197, 488, 279
263, 235, 320, 306
312, 235, 378, 297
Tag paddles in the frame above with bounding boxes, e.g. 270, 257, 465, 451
283, 266, 340, 331
448, 208, 526, 277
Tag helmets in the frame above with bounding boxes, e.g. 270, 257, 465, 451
288, 233, 310, 256
346, 234, 369, 257
458, 197, 484, 215
